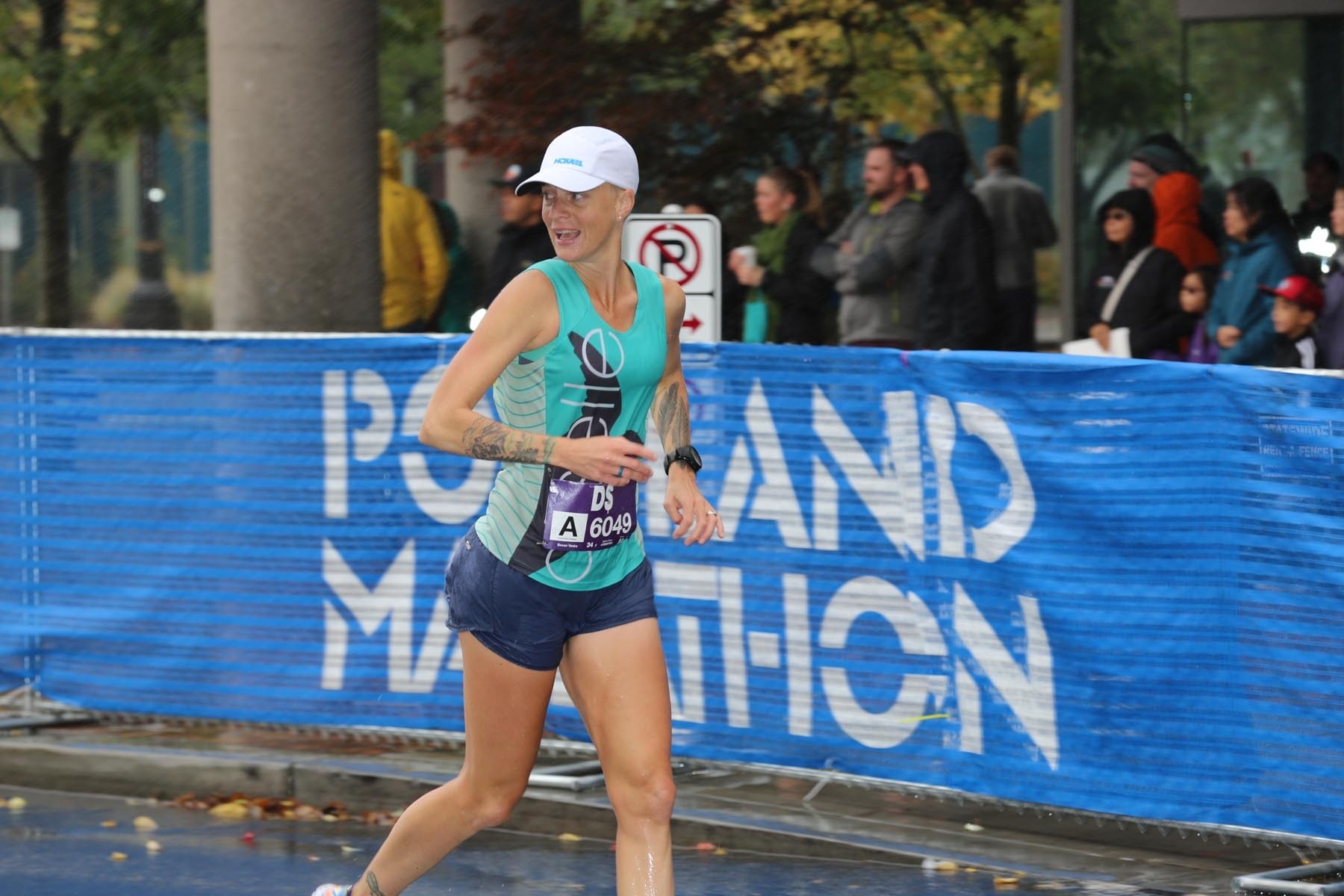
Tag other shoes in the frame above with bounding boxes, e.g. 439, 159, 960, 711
310, 883, 353, 896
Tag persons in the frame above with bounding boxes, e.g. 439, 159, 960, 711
311, 143, 720, 896
1072, 130, 1344, 372
377, 126, 1060, 353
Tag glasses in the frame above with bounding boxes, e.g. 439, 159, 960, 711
1103, 213, 1125, 221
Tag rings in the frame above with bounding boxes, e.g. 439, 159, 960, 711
618, 466, 624, 477
705, 510, 717, 516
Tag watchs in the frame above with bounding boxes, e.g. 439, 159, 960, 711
663, 442, 704, 475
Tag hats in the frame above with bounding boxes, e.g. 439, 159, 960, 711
515, 126, 639, 198
1257, 276, 1323, 316
488, 162, 537, 189
1128, 144, 1183, 174
